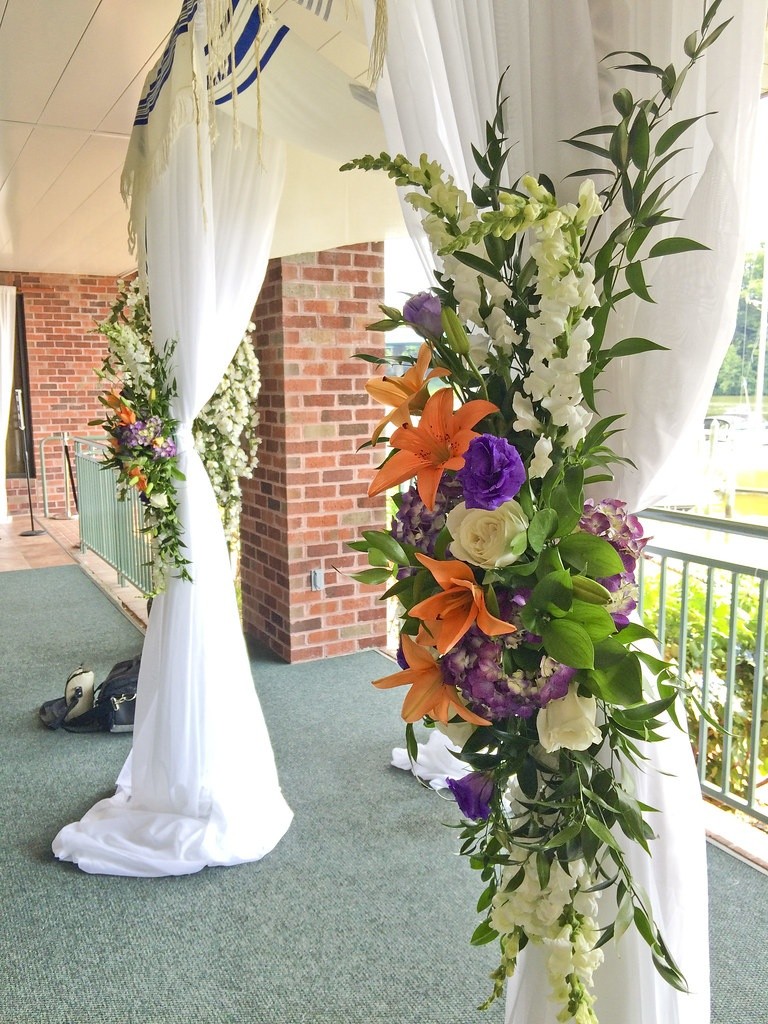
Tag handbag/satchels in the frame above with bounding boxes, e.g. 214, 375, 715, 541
51, 654, 142, 733
39, 664, 94, 730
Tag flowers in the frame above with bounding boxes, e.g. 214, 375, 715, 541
88, 270, 261, 585
337, 0, 736, 1024
